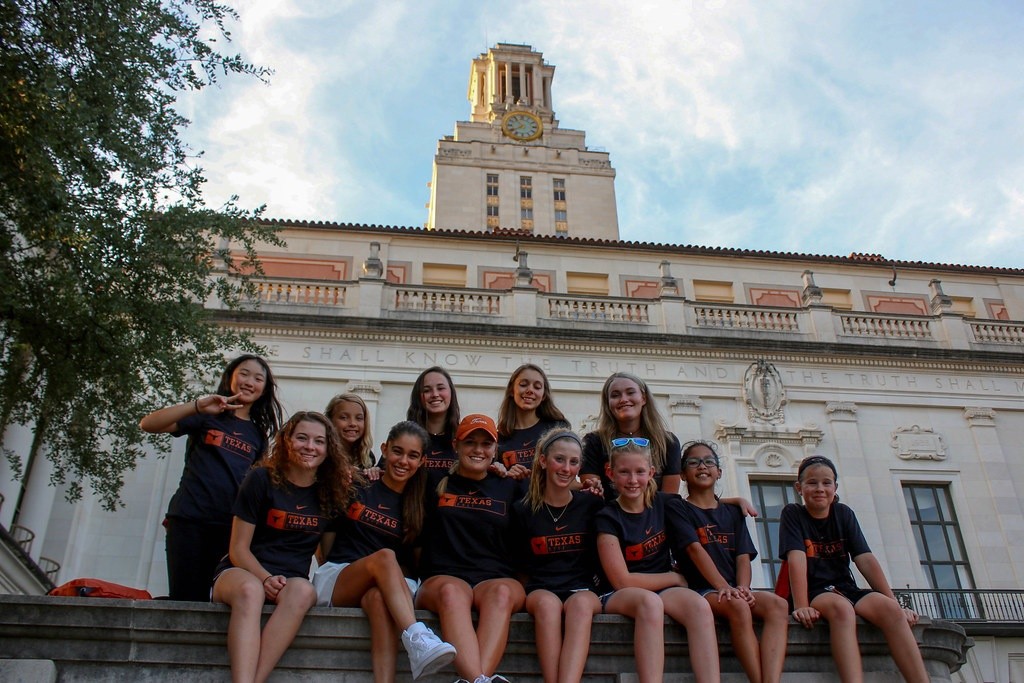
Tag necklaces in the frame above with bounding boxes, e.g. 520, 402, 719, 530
433, 429, 444, 436
618, 430, 638, 436
545, 497, 569, 523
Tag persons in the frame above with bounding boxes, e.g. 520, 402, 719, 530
311, 420, 458, 682
414, 414, 602, 682
511, 428, 604, 683
665, 439, 788, 683
363, 367, 508, 495
597, 437, 757, 682
140, 355, 283, 601
581, 373, 681, 494
773, 456, 931, 683
324, 393, 375, 474
208, 409, 365, 683
494, 364, 606, 497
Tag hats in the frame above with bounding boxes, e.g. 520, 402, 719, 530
456, 413, 498, 443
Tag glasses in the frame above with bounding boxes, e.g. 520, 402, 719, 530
610, 437, 651, 450
682, 457, 718, 471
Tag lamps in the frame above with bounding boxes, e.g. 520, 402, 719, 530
658, 260, 676, 288
512, 251, 533, 283
927, 279, 952, 311
801, 269, 823, 304
362, 240, 384, 276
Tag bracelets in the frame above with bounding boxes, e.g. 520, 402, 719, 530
264, 575, 272, 583
194, 399, 198, 412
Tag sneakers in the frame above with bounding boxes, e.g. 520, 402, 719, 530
460, 675, 511, 683
401, 621, 457, 681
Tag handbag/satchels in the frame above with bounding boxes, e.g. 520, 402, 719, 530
44, 578, 153, 600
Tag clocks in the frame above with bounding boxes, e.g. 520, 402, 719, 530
501, 110, 543, 142
